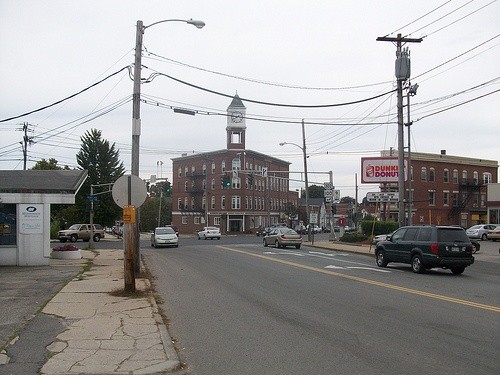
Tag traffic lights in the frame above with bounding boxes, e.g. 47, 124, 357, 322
221, 175, 231, 187
164, 182, 171, 194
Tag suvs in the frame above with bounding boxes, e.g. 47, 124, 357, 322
58, 224, 105, 242
374, 223, 475, 275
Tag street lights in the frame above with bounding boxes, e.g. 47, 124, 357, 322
131, 18, 206, 274
279, 141, 312, 241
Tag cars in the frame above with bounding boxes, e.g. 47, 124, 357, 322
150, 226, 179, 248
256, 224, 341, 236
197, 226, 222, 240
465, 224, 500, 254
262, 227, 302, 249
373, 230, 405, 243
105, 227, 112, 233
